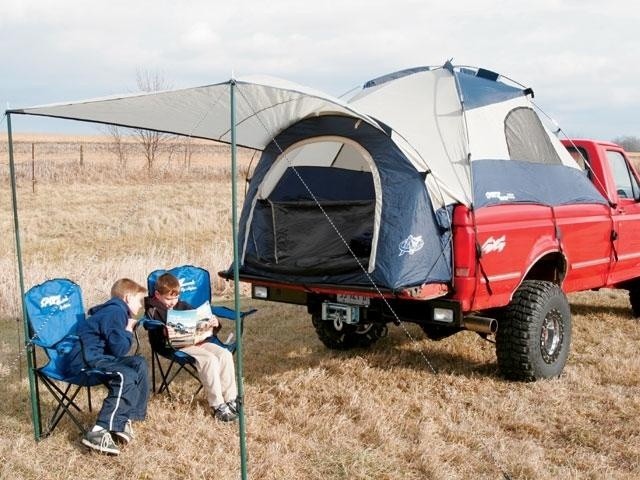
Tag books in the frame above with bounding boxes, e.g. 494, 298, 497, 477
166, 300, 214, 348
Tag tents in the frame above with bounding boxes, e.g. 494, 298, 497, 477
227, 62, 606, 290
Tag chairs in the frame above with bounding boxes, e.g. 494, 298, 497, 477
143, 265, 257, 398
24, 278, 145, 435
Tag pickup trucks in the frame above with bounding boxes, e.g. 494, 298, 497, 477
218, 60, 640, 382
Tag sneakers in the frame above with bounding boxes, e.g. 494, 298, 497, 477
227, 401, 239, 415
111, 420, 134, 444
81, 429, 120, 457
211, 404, 239, 423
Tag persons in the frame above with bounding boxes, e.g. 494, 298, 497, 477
144, 273, 238, 421
61, 278, 148, 457
